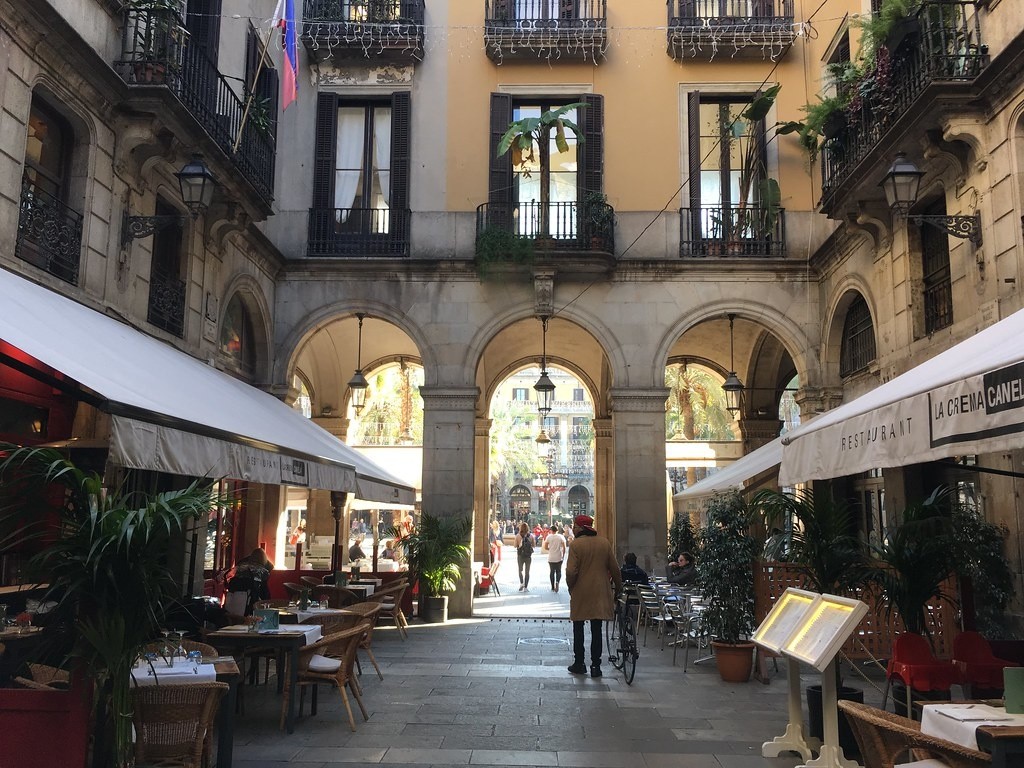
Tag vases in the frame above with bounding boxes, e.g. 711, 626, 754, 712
319, 600, 328, 610
248, 622, 260, 633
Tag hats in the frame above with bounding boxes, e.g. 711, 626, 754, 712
574, 514, 595, 532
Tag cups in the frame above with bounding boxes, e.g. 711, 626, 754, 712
142, 653, 158, 671
651, 571, 656, 581
1003, 667, 1024, 713
189, 651, 202, 666
351, 575, 356, 581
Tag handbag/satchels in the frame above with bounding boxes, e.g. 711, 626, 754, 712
496, 539, 503, 546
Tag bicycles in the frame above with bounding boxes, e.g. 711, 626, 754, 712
605, 577, 641, 684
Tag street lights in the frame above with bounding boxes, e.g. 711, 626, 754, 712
546, 453, 553, 526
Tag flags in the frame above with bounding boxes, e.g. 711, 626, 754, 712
271, 0, 300, 111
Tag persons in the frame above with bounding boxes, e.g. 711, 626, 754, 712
349, 540, 365, 559
513, 523, 537, 591
488, 510, 574, 546
380, 540, 395, 561
286, 526, 308, 545
762, 526, 784, 579
617, 553, 651, 587
566, 515, 624, 679
352, 517, 395, 534
544, 525, 565, 592
665, 552, 698, 587
227, 549, 271, 605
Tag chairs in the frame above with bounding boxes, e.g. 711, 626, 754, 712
950, 631, 1022, 700
0, 576, 409, 768
838, 700, 992, 768
880, 631, 955, 720
614, 574, 716, 675
480, 560, 501, 597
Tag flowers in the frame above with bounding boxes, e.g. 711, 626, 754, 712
318, 593, 329, 603
243, 615, 263, 632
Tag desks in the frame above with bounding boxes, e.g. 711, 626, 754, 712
274, 607, 352, 626
0, 583, 51, 619
347, 579, 383, 592
0, 626, 46, 689
206, 625, 324, 735
911, 701, 1024, 768
128, 656, 240, 768
317, 585, 374, 602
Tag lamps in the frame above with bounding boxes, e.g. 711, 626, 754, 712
721, 313, 744, 420
347, 312, 369, 415
877, 150, 982, 248
120, 153, 216, 263
533, 315, 556, 420
535, 417, 551, 444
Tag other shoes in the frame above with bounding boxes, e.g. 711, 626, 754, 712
568, 662, 588, 673
519, 583, 524, 591
556, 582, 559, 593
524, 588, 529, 592
552, 586, 554, 591
591, 669, 602, 677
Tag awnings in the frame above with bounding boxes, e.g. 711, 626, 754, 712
777, 308, 1024, 488
0, 266, 416, 505
672, 431, 800, 512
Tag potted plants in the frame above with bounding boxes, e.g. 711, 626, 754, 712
747, 481, 977, 765
710, 81, 819, 256
693, 484, 757, 683
129, 30, 165, 84
496, 103, 591, 250
847, 0, 923, 66
581, 192, 618, 251
377, 509, 475, 623
797, 94, 848, 148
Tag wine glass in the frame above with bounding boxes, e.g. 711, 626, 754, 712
160, 630, 189, 666
0, 604, 10, 632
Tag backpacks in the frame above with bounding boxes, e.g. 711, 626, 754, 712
519, 533, 532, 557
